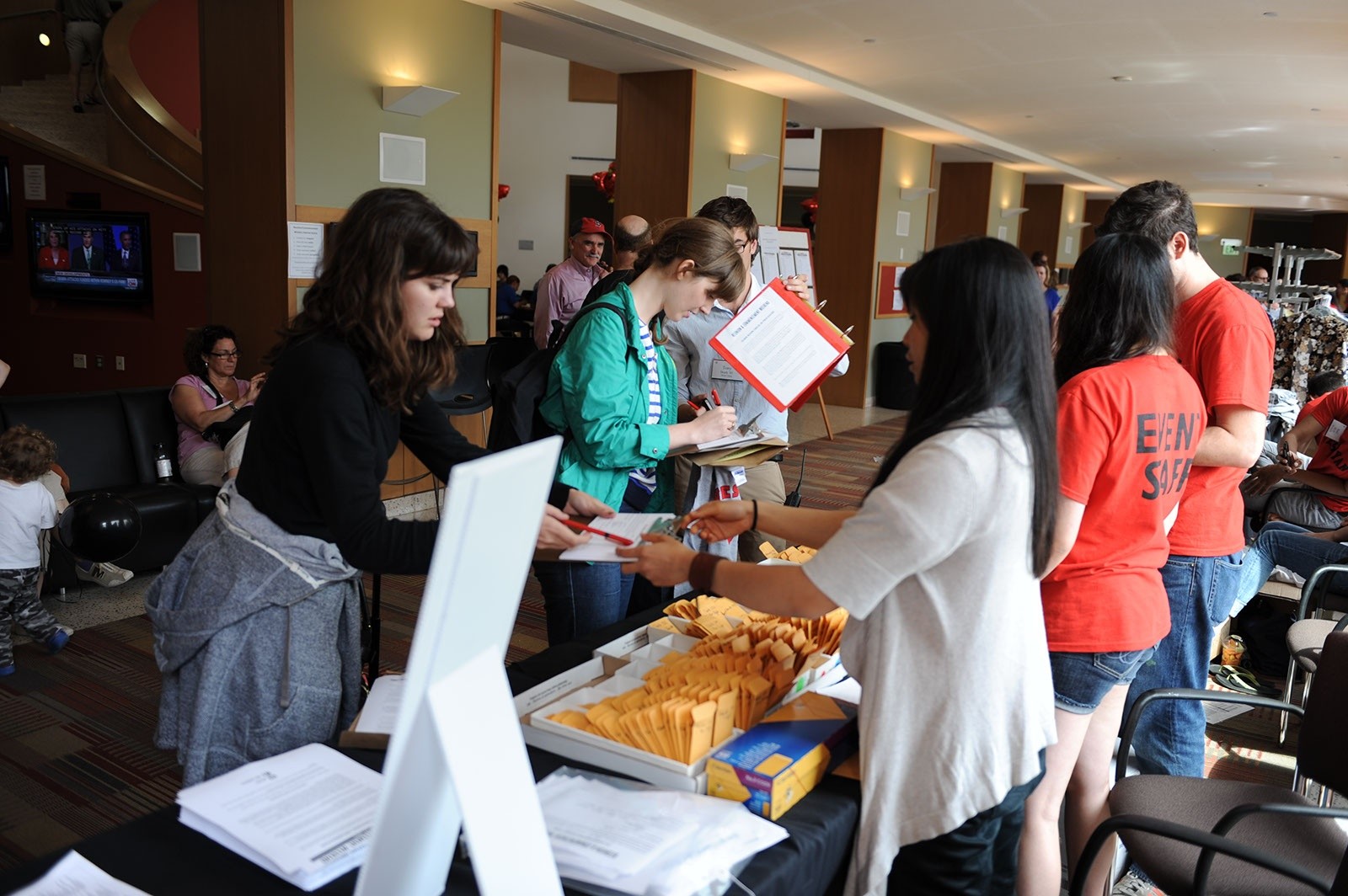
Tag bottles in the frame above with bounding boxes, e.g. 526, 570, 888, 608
156, 439, 175, 487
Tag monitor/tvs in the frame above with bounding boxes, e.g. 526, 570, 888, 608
24, 206, 154, 309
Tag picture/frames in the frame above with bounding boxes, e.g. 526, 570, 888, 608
875, 260, 915, 320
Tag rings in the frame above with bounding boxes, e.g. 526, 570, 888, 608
728, 421, 736, 430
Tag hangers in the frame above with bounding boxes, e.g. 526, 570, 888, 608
1243, 284, 1348, 331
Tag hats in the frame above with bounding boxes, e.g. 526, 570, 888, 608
569, 216, 613, 242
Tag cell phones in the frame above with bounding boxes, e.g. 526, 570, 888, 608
1283, 441, 1291, 473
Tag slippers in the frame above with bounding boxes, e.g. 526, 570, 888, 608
1214, 672, 1283, 697
1207, 665, 1276, 688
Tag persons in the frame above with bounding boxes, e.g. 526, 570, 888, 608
1213, 369, 1348, 637
1014, 232, 1208, 896
521, 217, 749, 641
142, 189, 613, 794
614, 236, 1059, 896
1221, 265, 1348, 317
39, 227, 141, 275
0, 359, 133, 599
1097, 179, 1276, 775
0, 424, 74, 674
660, 197, 850, 518
496, 215, 655, 351
1029, 252, 1059, 340
167, 322, 268, 490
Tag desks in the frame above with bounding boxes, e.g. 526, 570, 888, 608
3, 546, 921, 896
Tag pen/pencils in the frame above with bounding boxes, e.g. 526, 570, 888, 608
687, 400, 699, 411
560, 519, 633, 545
711, 389, 721, 406
701, 397, 713, 411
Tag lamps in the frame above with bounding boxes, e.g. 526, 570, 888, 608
382, 83, 460, 118
1197, 233, 1220, 243
1067, 222, 1091, 230
1001, 206, 1029, 217
899, 185, 936, 203
728, 152, 779, 173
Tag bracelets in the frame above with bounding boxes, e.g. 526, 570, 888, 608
687, 552, 730, 599
750, 499, 758, 532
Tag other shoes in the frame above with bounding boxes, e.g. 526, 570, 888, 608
0, 664, 16, 677
47, 628, 69, 655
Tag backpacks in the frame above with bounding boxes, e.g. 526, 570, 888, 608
480, 302, 631, 459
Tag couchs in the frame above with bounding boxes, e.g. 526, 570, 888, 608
1, 386, 227, 598
875, 341, 917, 411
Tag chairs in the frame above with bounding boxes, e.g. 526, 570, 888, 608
1275, 561, 1348, 745
1246, 394, 1347, 553
431, 341, 492, 450
1104, 612, 1348, 896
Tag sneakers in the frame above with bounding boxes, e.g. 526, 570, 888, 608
75, 558, 134, 588
1111, 869, 1153, 896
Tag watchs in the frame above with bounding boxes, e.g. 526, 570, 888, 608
227, 399, 239, 413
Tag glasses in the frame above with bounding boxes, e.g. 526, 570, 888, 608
734, 237, 750, 255
1254, 275, 1270, 281
202, 349, 243, 360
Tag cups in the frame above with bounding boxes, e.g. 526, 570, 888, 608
1220, 635, 1246, 666
